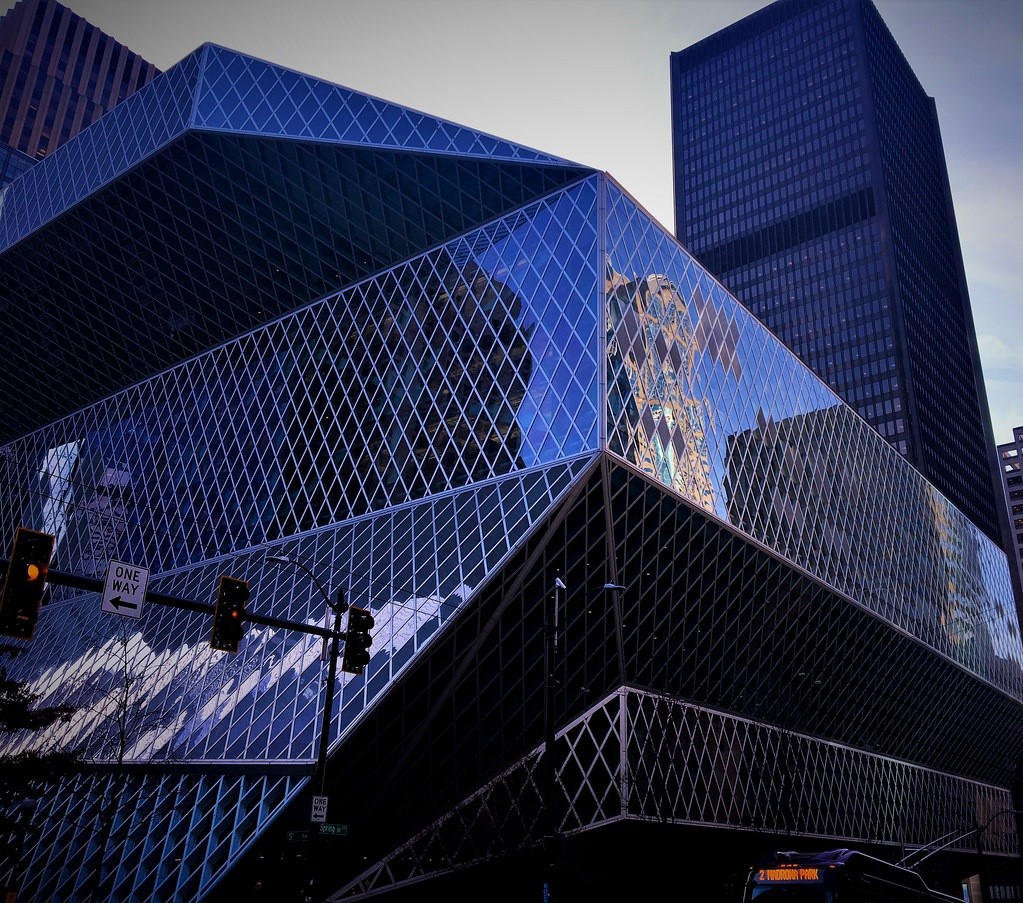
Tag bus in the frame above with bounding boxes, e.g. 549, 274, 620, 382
743, 829, 974, 903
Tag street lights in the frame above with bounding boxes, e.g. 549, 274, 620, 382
265, 556, 349, 903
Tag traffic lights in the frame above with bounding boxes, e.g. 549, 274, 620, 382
211, 576, 250, 653
0, 528, 56, 640
342, 607, 375, 675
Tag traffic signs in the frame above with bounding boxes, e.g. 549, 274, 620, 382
100, 559, 150, 620
311, 796, 328, 823
